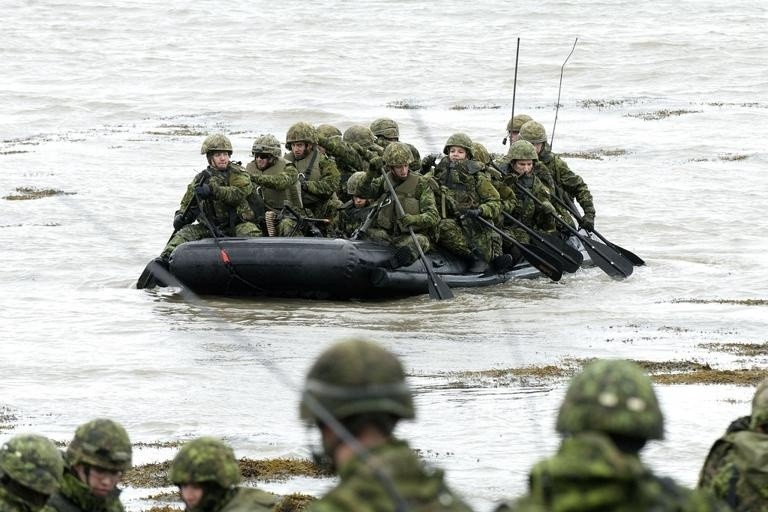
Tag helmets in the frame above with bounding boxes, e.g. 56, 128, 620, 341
507, 115, 532, 131
251, 135, 281, 158
68, 421, 132, 470
302, 341, 415, 418
286, 123, 319, 144
443, 134, 474, 158
519, 122, 547, 143
201, 134, 233, 154
348, 172, 368, 195
750, 380, 768, 424
343, 126, 376, 147
317, 124, 342, 150
506, 140, 539, 162
370, 119, 399, 138
556, 360, 665, 441
382, 143, 414, 166
472, 143, 490, 164
0, 433, 63, 494
171, 438, 240, 488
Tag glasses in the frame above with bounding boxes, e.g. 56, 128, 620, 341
255, 154, 268, 159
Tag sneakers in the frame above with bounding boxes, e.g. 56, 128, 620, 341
390, 245, 412, 267
494, 254, 510, 272
152, 259, 168, 287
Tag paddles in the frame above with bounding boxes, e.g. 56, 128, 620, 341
551, 194, 645, 266
490, 157, 633, 280
379, 166, 455, 300
476, 215, 564, 281
501, 210, 583, 273
137, 176, 207, 290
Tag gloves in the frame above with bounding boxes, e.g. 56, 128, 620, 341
505, 175, 518, 185
173, 215, 191, 229
422, 155, 436, 173
195, 183, 212, 198
460, 207, 481, 219
581, 216, 594, 232
400, 213, 417, 227
542, 201, 555, 214
368, 157, 384, 170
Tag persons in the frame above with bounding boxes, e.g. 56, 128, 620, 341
503, 113, 532, 148
40, 418, 132, 512
159, 134, 263, 259
342, 124, 384, 165
420, 133, 514, 273
314, 124, 364, 201
168, 438, 276, 512
0, 435, 64, 512
469, 142, 527, 265
246, 135, 303, 237
701, 377, 768, 512
355, 142, 441, 270
497, 140, 557, 238
329, 169, 380, 241
299, 338, 472, 512
370, 118, 398, 146
281, 121, 344, 237
514, 120, 596, 233
490, 360, 735, 512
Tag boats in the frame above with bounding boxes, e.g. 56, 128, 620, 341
167, 229, 594, 296
